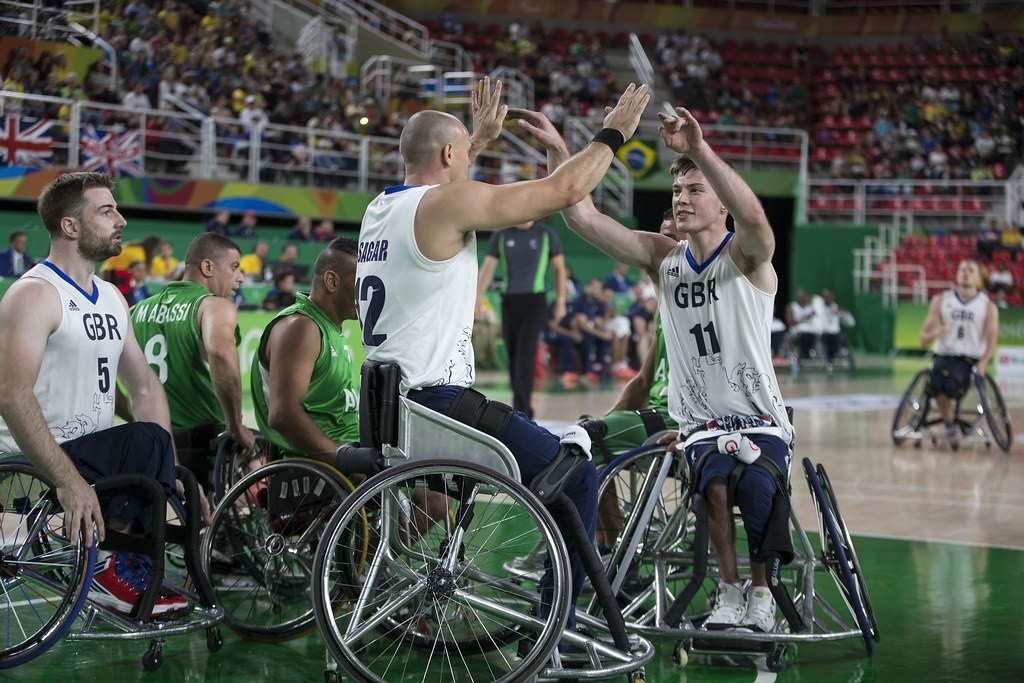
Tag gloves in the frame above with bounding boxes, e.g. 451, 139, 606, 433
335, 443, 382, 475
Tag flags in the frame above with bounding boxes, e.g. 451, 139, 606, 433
80, 123, 141, 178
0, 110, 56, 167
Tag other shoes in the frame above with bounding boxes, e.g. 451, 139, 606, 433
583, 552, 639, 587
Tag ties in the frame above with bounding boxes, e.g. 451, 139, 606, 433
16, 257, 24, 276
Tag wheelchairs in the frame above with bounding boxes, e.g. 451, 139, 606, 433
890, 367, 1012, 456
586, 440, 879, 669
202, 434, 411, 641
0, 454, 221, 673
306, 362, 652, 683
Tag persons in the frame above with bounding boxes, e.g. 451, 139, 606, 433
473, 263, 658, 389
770, 285, 857, 363
438, 0, 611, 74
0, 170, 212, 616
473, 217, 567, 420
115, 232, 453, 610
388, 70, 427, 118
0, 207, 340, 310
976, 219, 1024, 310
657, 31, 1024, 189
355, 75, 651, 662
0, 0, 383, 186
921, 259, 999, 452
517, 107, 796, 635
575, 324, 747, 629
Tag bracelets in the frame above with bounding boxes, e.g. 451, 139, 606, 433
592, 129, 624, 155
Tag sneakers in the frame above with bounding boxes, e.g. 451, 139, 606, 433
741, 589, 775, 633
704, 581, 748, 629
84, 553, 194, 621
